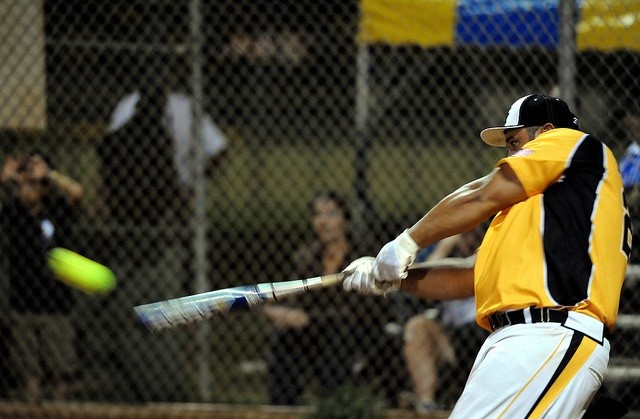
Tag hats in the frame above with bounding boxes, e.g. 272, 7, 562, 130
480, 92, 579, 147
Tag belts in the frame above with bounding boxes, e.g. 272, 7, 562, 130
488, 307, 611, 342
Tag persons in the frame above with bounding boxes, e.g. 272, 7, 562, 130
96, 78, 229, 380
266, 190, 384, 404
401, 208, 495, 418
0, 136, 85, 403
616, 97, 640, 279
341, 92, 632, 419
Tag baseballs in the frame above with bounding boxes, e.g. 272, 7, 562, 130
46, 247, 116, 298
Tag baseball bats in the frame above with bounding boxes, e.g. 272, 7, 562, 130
133, 272, 348, 335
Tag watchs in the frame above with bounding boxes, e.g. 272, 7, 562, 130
39, 170, 59, 188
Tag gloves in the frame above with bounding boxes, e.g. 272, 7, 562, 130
342, 256, 401, 298
371, 227, 423, 283
